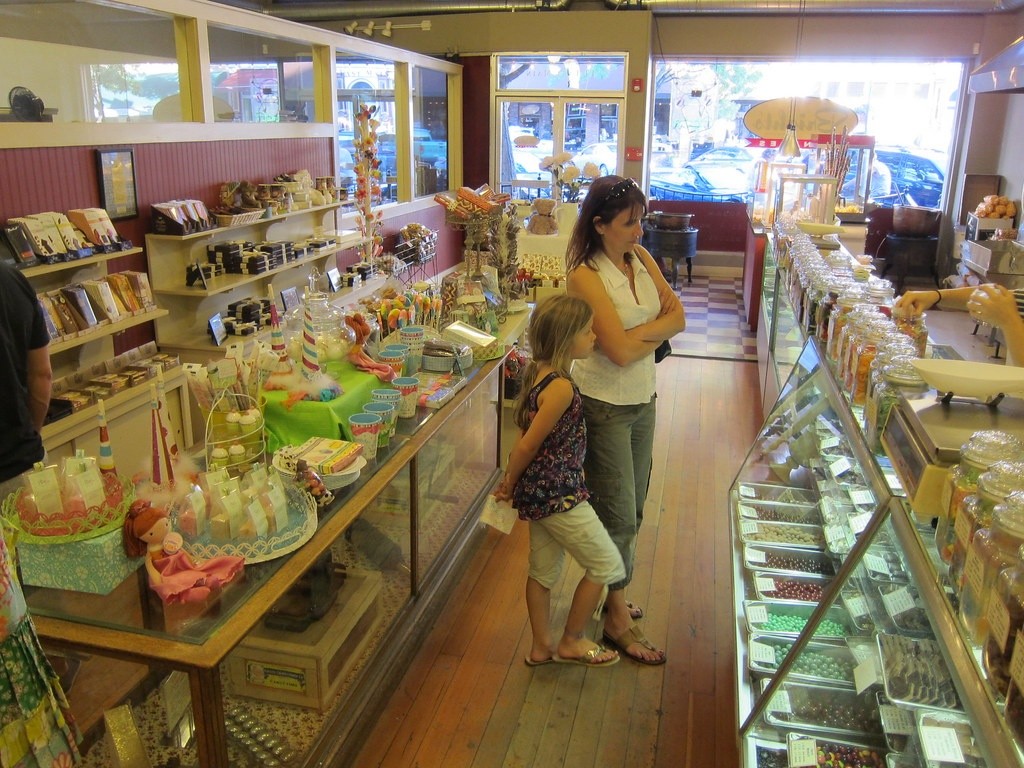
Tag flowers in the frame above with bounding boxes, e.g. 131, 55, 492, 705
539, 152, 600, 202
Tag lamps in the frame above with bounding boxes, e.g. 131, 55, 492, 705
777, 1, 808, 156
382, 19, 392, 37
362, 21, 375, 36
343, 20, 358, 34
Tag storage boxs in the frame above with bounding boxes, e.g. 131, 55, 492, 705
229, 571, 386, 710
19, 526, 145, 596
443, 319, 498, 356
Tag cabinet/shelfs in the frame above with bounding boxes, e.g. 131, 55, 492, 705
18, 248, 196, 483
144, 195, 368, 369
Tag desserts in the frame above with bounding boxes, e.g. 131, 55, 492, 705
211, 440, 245, 466
225, 405, 260, 435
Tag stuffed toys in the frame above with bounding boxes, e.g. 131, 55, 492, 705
527, 198, 558, 236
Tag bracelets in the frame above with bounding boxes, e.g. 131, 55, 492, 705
933, 290, 941, 305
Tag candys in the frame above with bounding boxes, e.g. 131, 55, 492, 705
741, 492, 830, 601
775, 698, 881, 732
374, 288, 442, 328
754, 613, 857, 682
353, 104, 383, 255
816, 743, 884, 768
939, 465, 1022, 644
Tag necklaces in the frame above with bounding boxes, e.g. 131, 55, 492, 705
616, 261, 629, 278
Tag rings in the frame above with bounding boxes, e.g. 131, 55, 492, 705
661, 311, 666, 314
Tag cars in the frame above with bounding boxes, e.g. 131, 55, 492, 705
335, 126, 448, 190
511, 124, 946, 213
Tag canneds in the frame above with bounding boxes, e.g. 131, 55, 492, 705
314, 176, 348, 203
257, 183, 291, 216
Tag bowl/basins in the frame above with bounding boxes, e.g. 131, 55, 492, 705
893, 204, 942, 237
655, 212, 694, 230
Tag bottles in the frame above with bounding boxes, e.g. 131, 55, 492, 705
935, 430, 1024, 748
772, 210, 929, 454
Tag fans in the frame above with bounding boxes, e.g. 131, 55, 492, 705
10, 87, 44, 122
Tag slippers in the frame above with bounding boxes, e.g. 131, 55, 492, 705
602, 622, 667, 665
598, 600, 643, 619
552, 642, 620, 668
524, 651, 554, 666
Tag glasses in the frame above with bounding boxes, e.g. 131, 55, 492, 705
593, 177, 639, 214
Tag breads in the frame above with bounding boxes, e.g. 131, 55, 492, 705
815, 441, 927, 630
180, 486, 276, 539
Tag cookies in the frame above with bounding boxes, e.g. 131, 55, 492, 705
878, 632, 957, 710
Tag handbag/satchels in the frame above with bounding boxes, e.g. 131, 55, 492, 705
629, 244, 672, 363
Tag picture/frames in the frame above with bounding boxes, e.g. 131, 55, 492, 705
94, 148, 141, 221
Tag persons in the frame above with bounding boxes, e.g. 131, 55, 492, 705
566, 175, 685, 663
0, 259, 52, 503
895, 279, 1024, 368
842, 149, 891, 205
492, 293, 627, 667
119, 497, 246, 605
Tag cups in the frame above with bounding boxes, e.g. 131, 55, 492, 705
378, 326, 424, 378
349, 413, 381, 458
371, 389, 401, 438
392, 377, 419, 418
362, 402, 394, 447
